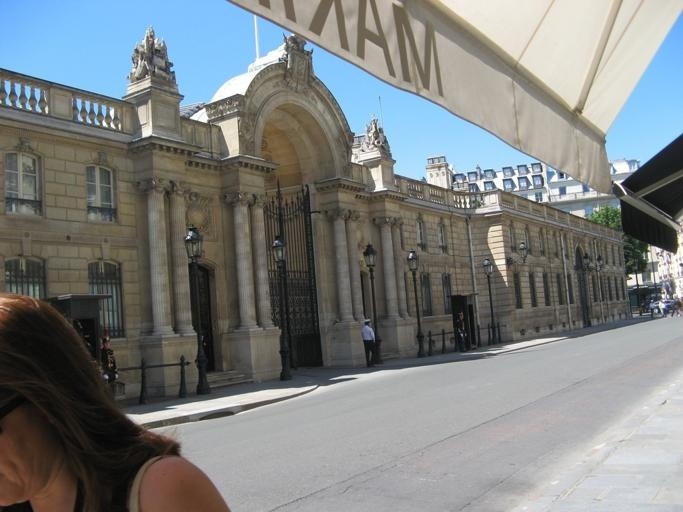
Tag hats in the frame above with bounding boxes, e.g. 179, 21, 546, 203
362, 318, 371, 325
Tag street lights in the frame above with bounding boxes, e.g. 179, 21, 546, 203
408, 250, 425, 357
271, 240, 290, 380
185, 226, 209, 394
483, 258, 497, 345
362, 245, 382, 364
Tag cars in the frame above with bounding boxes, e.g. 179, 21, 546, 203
642, 299, 674, 313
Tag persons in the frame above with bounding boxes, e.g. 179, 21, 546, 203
99, 334, 119, 402
454, 310, 467, 352
1, 291, 233, 512
361, 317, 377, 368
648, 300, 655, 319
658, 299, 664, 318
670, 294, 681, 317
